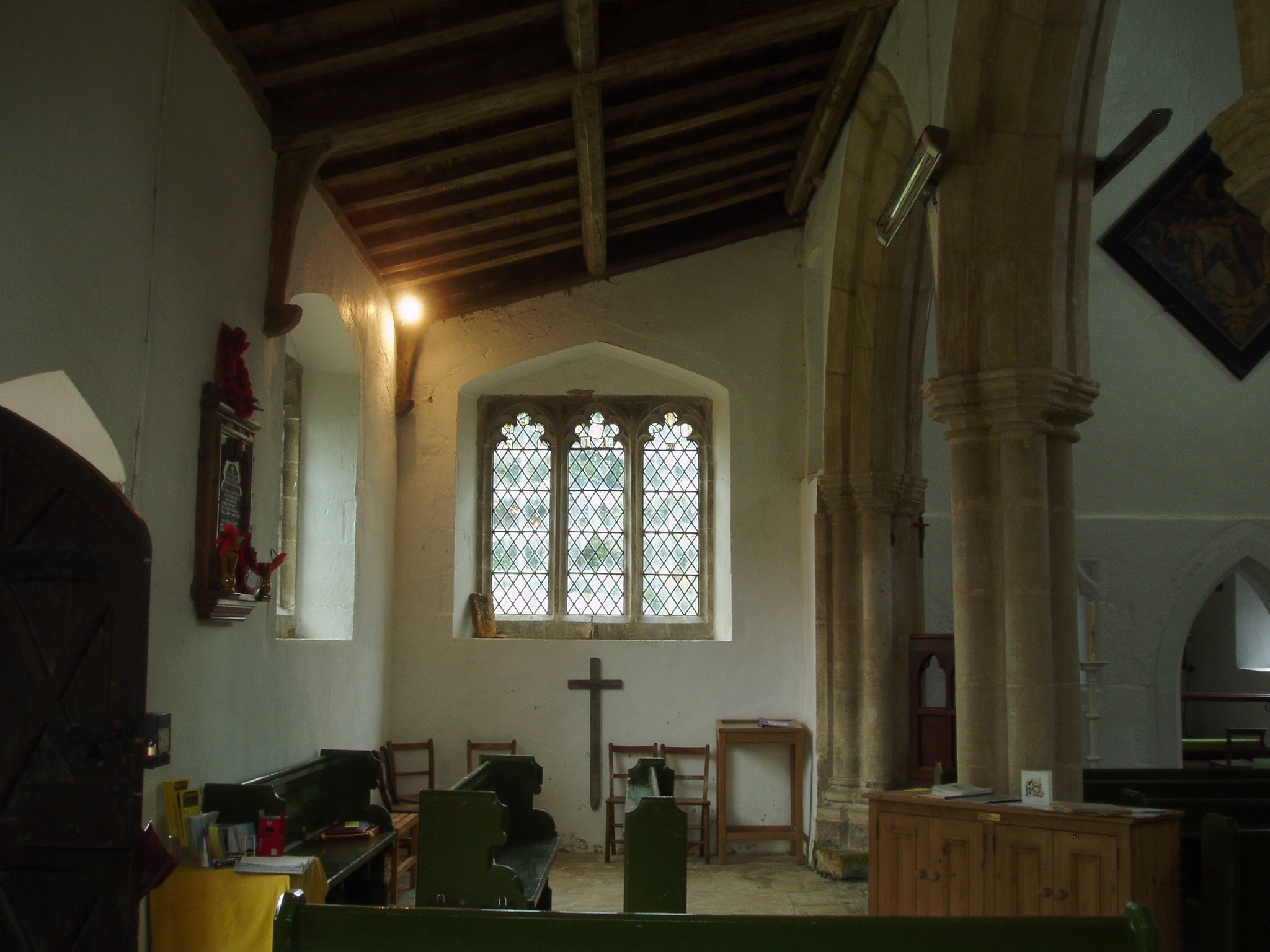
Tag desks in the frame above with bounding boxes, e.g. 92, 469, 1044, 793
715, 718, 806, 866
154, 857, 319, 952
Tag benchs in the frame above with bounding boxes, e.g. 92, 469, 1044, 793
416, 753, 562, 910
148, 829, 397, 952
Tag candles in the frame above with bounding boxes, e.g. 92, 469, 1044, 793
1085, 596, 1096, 660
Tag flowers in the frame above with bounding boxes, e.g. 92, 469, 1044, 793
213, 523, 287, 577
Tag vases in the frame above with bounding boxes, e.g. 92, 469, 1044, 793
217, 552, 238, 593
255, 563, 273, 599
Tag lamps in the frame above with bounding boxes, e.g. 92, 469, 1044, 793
870, 122, 951, 251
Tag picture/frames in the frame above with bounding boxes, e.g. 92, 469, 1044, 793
1094, 126, 1270, 381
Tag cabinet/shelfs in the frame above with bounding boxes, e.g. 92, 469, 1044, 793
907, 633, 955, 782
861, 788, 1183, 952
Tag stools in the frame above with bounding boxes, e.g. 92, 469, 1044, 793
388, 813, 419, 904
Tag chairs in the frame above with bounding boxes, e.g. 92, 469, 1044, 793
660, 743, 712, 864
386, 739, 434, 805
373, 746, 419, 811
466, 739, 516, 776
604, 742, 658, 864
